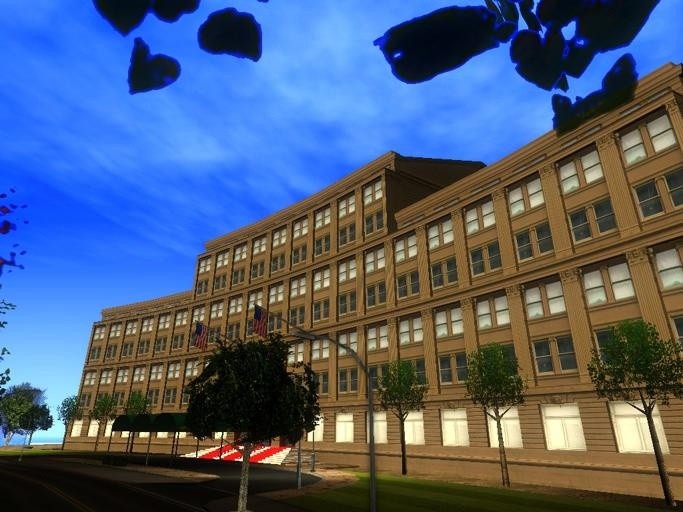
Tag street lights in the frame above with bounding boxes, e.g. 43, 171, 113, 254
292, 330, 375, 511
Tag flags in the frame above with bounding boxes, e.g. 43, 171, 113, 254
190, 321, 210, 352
251, 304, 269, 338
